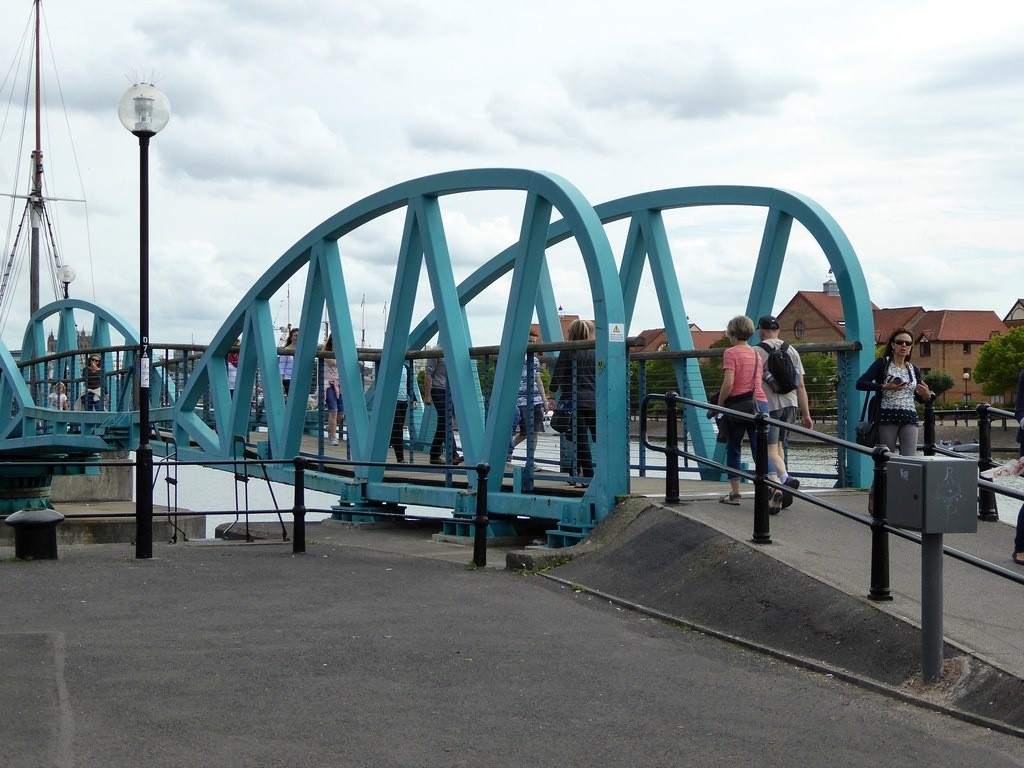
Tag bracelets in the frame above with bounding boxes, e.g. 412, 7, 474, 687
549, 397, 555, 399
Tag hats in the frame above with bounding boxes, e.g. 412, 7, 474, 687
755, 316, 779, 330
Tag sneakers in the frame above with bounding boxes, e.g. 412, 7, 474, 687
782, 477, 799, 507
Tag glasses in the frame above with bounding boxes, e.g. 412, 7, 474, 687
894, 340, 912, 346
92, 358, 99, 362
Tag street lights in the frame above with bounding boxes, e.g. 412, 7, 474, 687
56, 264, 77, 411
963, 372, 971, 428
116, 81, 170, 559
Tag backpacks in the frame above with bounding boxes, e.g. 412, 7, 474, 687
755, 341, 798, 394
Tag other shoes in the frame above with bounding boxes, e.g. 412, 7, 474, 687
430, 458, 444, 465
453, 456, 463, 466
534, 466, 542, 472
332, 438, 338, 445
1012, 552, 1024, 564
506, 448, 513, 463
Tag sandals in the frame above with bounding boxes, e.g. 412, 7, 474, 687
868, 498, 873, 515
575, 483, 589, 488
719, 492, 741, 504
769, 490, 782, 513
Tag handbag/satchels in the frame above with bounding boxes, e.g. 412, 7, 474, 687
725, 391, 759, 428
551, 407, 571, 433
855, 420, 879, 448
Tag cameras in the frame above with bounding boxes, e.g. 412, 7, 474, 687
887, 376, 904, 386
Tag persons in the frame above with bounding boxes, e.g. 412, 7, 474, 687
317, 333, 345, 446
425, 343, 464, 465
719, 316, 755, 505
549, 320, 596, 488
953, 403, 960, 426
227, 338, 240, 399
954, 439, 962, 445
43, 382, 69, 411
963, 404, 969, 426
938, 405, 945, 426
82, 354, 109, 411
1011, 369, 1024, 565
279, 328, 298, 396
856, 329, 936, 524
391, 363, 418, 463
939, 437, 948, 445
974, 439, 979, 444
752, 317, 814, 508
506, 329, 548, 472
